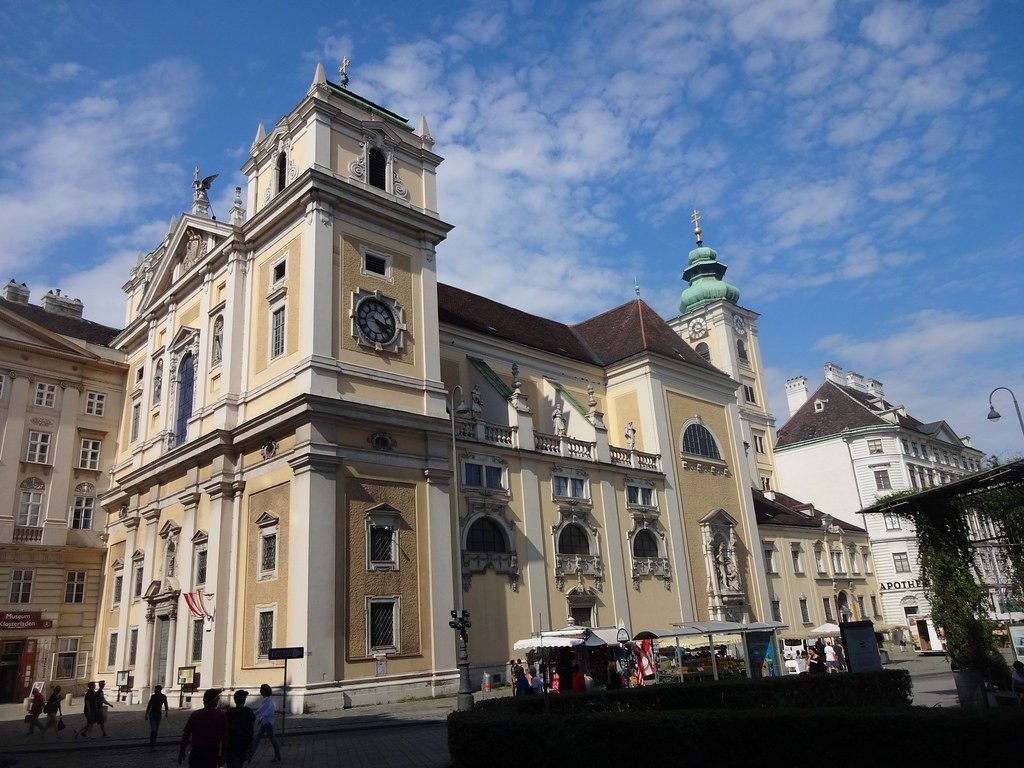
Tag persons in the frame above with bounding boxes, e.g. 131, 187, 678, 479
895, 627, 908, 652
807, 638, 847, 673
625, 421, 636, 449
178, 684, 282, 768
551, 404, 567, 435
510, 659, 594, 696
471, 384, 482, 418
24, 681, 113, 740
145, 685, 168, 752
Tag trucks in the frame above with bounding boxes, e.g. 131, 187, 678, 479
907, 612, 948, 655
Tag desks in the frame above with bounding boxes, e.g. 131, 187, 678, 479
658, 672, 700, 682
700, 671, 721, 680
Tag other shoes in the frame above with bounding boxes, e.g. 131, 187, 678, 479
88, 735, 96, 740
81, 732, 89, 738
150, 749, 158, 754
102, 732, 111, 738
270, 756, 282, 763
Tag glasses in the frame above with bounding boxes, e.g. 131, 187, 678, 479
101, 684, 106, 686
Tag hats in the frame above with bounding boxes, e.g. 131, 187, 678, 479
234, 690, 249, 700
88, 682, 97, 687
203, 688, 223, 701
32, 687, 39, 695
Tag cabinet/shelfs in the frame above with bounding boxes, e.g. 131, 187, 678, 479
655, 645, 728, 670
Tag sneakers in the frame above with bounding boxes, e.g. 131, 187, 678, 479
74, 729, 80, 740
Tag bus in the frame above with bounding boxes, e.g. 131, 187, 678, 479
782, 637, 807, 661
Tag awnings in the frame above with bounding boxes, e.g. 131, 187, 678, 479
580, 629, 628, 647
776, 623, 842, 640
513, 637, 584, 651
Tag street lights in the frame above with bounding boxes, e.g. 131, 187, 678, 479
987, 387, 1024, 438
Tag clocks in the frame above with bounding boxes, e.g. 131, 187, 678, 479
355, 296, 400, 344
733, 315, 746, 335
688, 318, 707, 339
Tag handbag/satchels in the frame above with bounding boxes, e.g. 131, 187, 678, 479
100, 706, 108, 722
254, 719, 262, 727
57, 717, 66, 731
24, 712, 34, 723
583, 673, 595, 693
